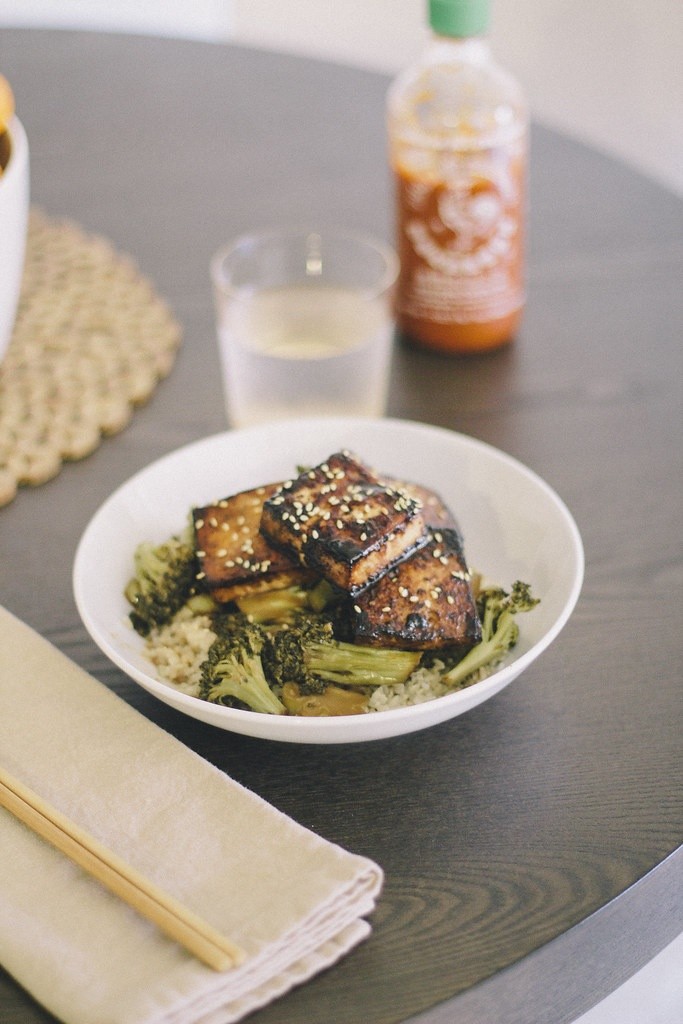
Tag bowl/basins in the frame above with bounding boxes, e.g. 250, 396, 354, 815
0, 115, 29, 365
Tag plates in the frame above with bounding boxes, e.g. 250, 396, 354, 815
73, 416, 587, 746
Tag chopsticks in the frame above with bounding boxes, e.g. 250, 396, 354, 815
0, 767, 249, 974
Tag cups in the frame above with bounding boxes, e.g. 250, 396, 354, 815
208, 226, 401, 428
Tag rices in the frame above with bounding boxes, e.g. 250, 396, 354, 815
144, 606, 479, 712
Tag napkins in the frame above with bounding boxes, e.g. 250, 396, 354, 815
0, 609, 384, 1024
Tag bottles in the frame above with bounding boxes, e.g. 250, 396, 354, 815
383, 0, 531, 355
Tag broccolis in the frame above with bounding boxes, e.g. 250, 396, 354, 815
128, 523, 543, 714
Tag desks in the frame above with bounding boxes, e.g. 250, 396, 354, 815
0, 28, 683, 1024
